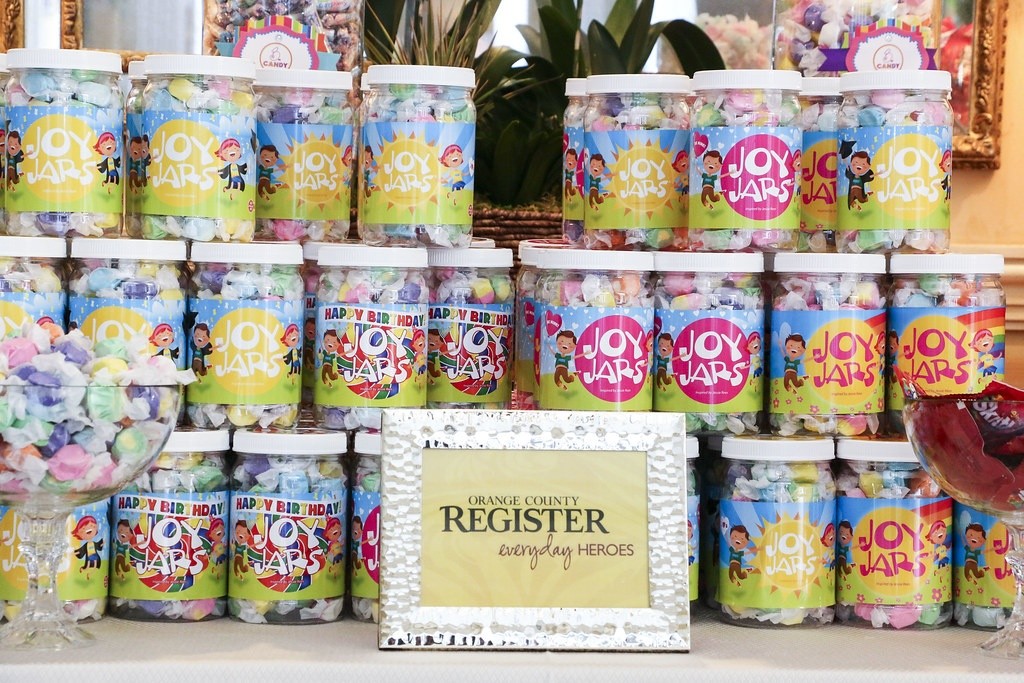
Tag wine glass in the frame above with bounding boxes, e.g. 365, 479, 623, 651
0, 385, 180, 654
902, 391, 1024, 659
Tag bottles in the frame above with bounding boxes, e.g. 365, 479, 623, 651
1, 50, 1024, 632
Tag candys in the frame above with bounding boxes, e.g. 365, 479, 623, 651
0, 0, 1024, 630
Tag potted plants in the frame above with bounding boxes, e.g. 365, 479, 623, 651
363, 0, 728, 280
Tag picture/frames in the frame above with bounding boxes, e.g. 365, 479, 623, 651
379, 409, 691, 651
940, 0, 1007, 169
